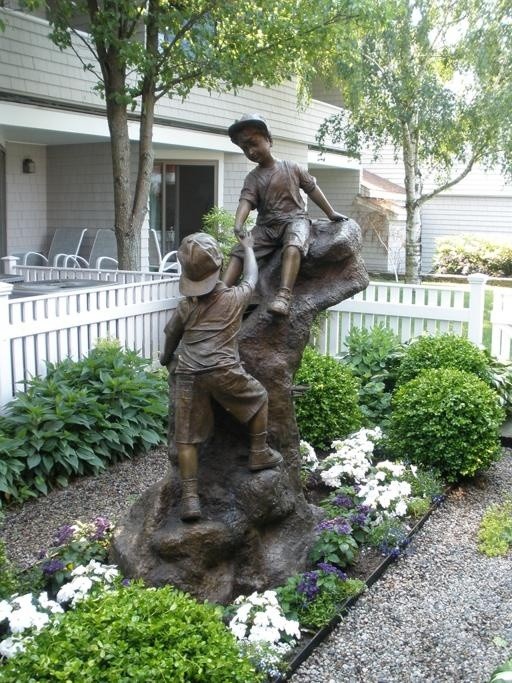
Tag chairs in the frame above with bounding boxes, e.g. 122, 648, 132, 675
21, 225, 90, 269
148, 227, 184, 275
62, 227, 119, 270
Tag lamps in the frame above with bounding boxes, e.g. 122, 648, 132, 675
23, 157, 37, 174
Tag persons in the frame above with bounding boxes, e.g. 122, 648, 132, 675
219, 114, 350, 315
159, 230, 284, 521
